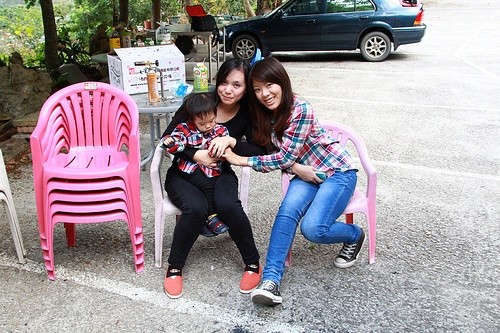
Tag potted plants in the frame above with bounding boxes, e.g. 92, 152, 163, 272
137, 15, 243, 31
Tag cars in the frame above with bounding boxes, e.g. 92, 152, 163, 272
210, 0, 426, 61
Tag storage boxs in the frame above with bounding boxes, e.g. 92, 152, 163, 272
107, 44, 186, 94
186, 5, 216, 32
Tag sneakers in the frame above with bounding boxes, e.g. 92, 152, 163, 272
207, 216, 229, 234
200, 224, 215, 237
251, 280, 282, 306
334, 228, 366, 268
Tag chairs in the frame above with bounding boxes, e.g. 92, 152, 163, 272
150, 136, 250, 268
281, 121, 377, 267
0, 81, 145, 280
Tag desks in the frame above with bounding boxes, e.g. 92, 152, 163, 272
156, 24, 226, 85
90, 53, 111, 63
130, 83, 216, 172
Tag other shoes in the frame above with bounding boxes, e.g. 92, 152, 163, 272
240, 261, 262, 294
164, 265, 182, 299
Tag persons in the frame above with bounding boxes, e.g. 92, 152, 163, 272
221, 58, 366, 306
159, 59, 272, 299
89, 24, 110, 57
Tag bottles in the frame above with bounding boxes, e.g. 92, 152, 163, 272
157, 20, 173, 46
146, 69, 159, 105
193, 63, 209, 92
